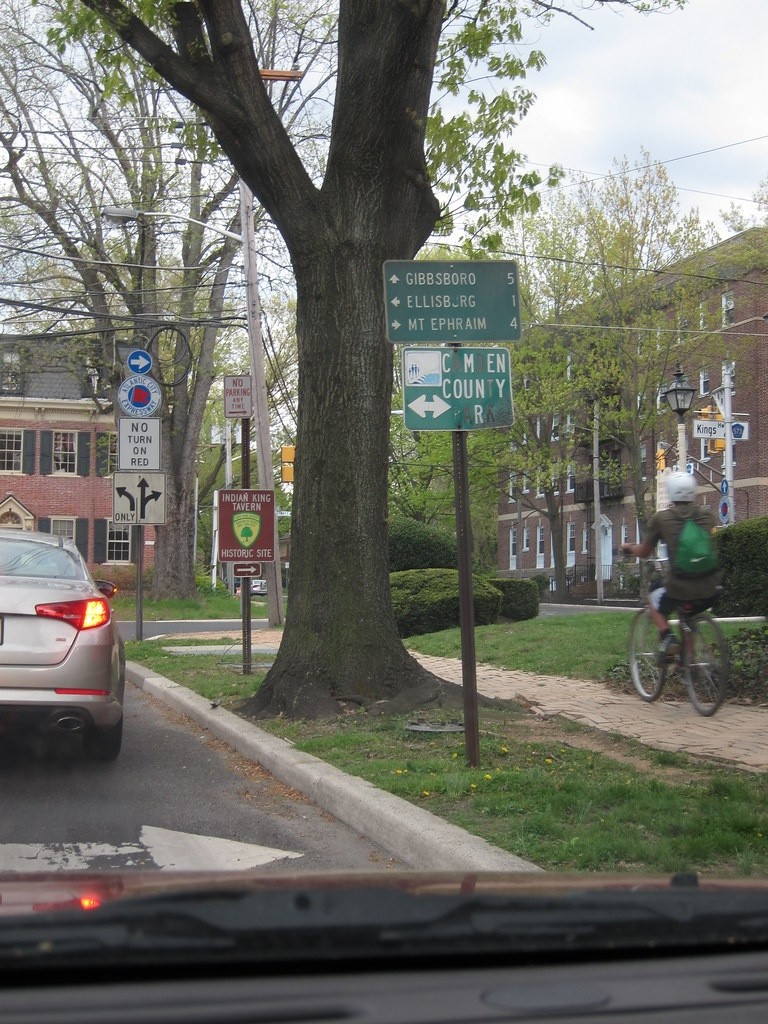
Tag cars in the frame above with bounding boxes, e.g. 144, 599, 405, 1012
0, 529, 126, 762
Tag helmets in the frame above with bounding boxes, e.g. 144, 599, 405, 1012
669, 473, 697, 502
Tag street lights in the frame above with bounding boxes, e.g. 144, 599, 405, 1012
664, 365, 697, 470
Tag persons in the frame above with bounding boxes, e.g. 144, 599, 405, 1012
622, 468, 720, 688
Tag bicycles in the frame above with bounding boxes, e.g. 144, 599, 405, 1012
613, 532, 733, 717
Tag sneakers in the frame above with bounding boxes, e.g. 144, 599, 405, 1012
655, 632, 681, 668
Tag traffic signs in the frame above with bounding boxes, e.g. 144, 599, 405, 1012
224, 373, 254, 420
215, 489, 277, 577
400, 347, 515, 432
381, 258, 525, 344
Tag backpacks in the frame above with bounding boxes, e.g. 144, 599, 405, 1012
674, 520, 719, 581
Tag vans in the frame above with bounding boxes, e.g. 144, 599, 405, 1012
0, 867, 767, 1022
